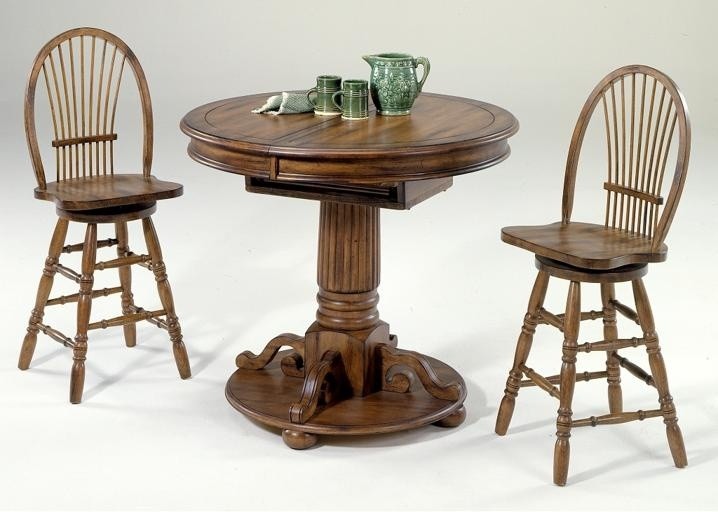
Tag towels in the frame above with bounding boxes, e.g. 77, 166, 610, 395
251, 89, 317, 117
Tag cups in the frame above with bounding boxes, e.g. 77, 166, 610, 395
306, 75, 342, 116
330, 79, 370, 122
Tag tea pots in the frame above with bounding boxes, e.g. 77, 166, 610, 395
363, 53, 430, 117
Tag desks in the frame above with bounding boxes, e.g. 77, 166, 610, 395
179, 88, 520, 452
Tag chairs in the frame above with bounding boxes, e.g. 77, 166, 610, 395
16, 24, 191, 406
499, 63, 692, 485
